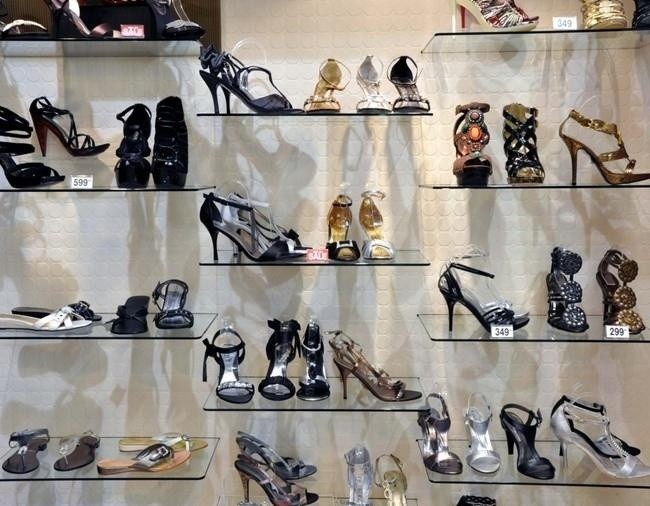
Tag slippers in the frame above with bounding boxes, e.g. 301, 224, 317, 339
118, 432, 208, 452
111, 296, 149, 334
53, 430, 101, 472
2, 428, 50, 474
97, 443, 192, 475
12, 301, 101, 321
0, 304, 92, 331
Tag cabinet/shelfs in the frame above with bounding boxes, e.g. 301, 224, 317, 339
1, 33, 222, 490
417, 23, 649, 494
190, 107, 434, 506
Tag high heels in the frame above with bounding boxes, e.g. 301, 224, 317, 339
216, 178, 313, 256
503, 103, 545, 183
558, 109, 650, 184
220, 87, 238, 115
551, 401, 650, 478
550, 395, 641, 458
596, 249, 646, 334
461, 0, 539, 36
235, 431, 317, 481
199, 192, 308, 261
387, 56, 430, 112
153, 280, 194, 329
456, 0, 540, 32
235, 454, 318, 506
296, 319, 330, 402
417, 393, 463, 475
198, 44, 303, 114
0, 106, 65, 188
29, 96, 110, 157
546, 246, 589, 333
500, 403, 555, 480
45, 1, 113, 38
359, 189, 394, 260
302, 58, 352, 113
202, 328, 254, 404
581, 0, 626, 30
258, 318, 302, 401
141, 1, 206, 38
438, 263, 529, 332
463, 391, 500, 474
355, 54, 393, 113
452, 102, 492, 185
325, 194, 361, 261
439, 244, 529, 318
333, 344, 422, 401
324, 330, 362, 381
630, 1, 650, 27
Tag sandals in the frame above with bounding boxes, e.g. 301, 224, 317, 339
373, 454, 408, 506
151, 96, 188, 189
0, 1, 49, 41
114, 103, 152, 188
344, 445, 373, 505
